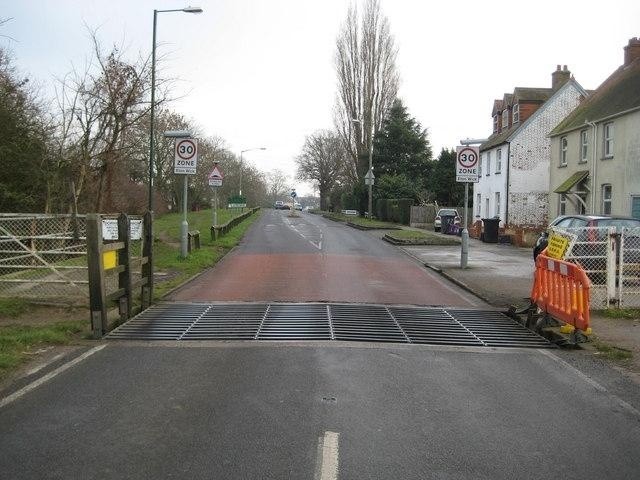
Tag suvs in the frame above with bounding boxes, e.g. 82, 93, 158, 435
533, 214, 640, 286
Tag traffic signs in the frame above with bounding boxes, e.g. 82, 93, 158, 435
455, 146, 480, 183
173, 137, 198, 176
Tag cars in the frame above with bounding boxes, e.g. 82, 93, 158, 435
341, 208, 361, 217
434, 208, 464, 233
275, 201, 320, 212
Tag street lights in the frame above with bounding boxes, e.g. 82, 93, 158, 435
351, 119, 373, 221
239, 147, 267, 202
147, 5, 204, 247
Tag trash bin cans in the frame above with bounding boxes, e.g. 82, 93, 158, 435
481, 218, 501, 243
